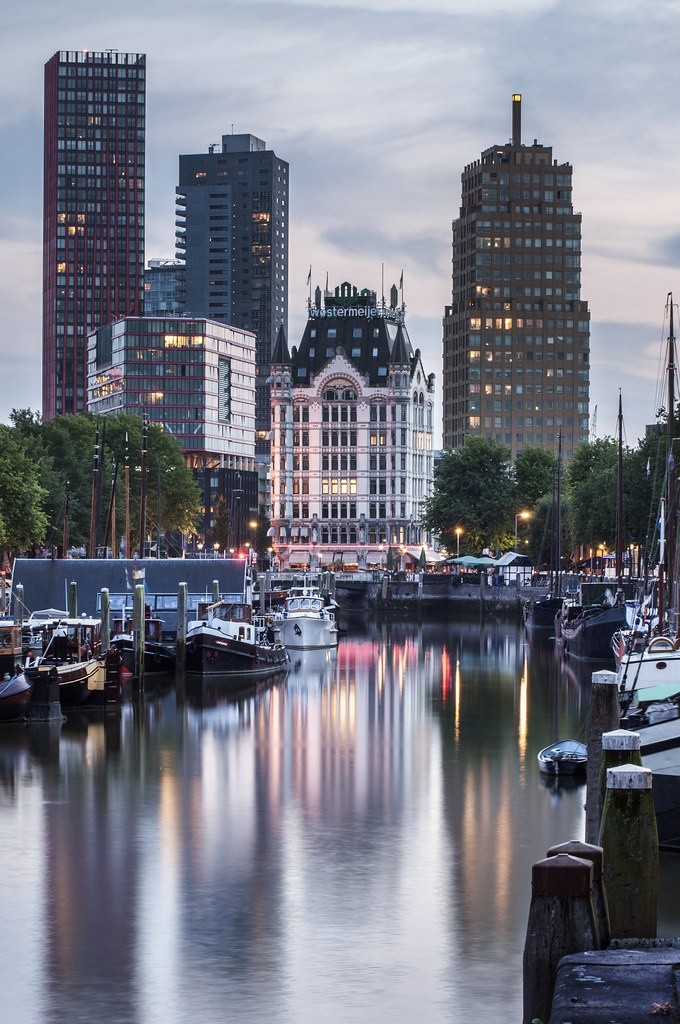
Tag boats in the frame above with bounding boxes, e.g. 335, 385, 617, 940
0, 587, 340, 717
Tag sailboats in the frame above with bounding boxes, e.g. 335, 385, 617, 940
522, 291, 680, 857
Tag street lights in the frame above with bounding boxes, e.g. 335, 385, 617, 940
454, 529, 463, 576
249, 520, 257, 551
514, 511, 530, 554
